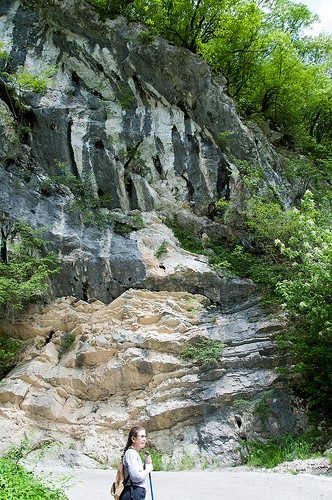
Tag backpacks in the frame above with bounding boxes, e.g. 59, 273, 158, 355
110, 447, 139, 500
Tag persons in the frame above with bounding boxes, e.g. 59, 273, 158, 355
118, 426, 153, 500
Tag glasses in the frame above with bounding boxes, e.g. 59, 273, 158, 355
137, 435, 147, 438
